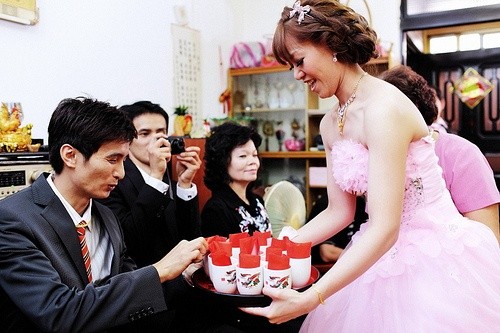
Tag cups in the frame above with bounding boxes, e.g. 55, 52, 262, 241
210, 261, 237, 294
298, 81, 305, 91
237, 264, 264, 294
290, 254, 311, 289
264, 264, 293, 289
203, 238, 287, 275
309, 167, 327, 185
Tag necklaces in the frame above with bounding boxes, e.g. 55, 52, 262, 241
336, 72, 365, 136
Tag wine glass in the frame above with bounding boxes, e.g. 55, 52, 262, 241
288, 81, 297, 107
273, 82, 284, 107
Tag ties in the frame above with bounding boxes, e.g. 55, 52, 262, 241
76, 221, 97, 284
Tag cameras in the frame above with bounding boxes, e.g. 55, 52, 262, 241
156, 136, 186, 156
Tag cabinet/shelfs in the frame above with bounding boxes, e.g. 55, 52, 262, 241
228, 54, 393, 218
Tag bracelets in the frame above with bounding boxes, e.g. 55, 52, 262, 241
311, 284, 324, 303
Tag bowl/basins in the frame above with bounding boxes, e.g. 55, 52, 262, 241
284, 139, 305, 151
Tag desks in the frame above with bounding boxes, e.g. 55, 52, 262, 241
169, 138, 211, 213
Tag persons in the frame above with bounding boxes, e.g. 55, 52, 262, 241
306, 187, 370, 265
199, 121, 272, 238
377, 65, 500, 243
100, 101, 202, 307
239, 0, 500, 333
0, 96, 208, 333
